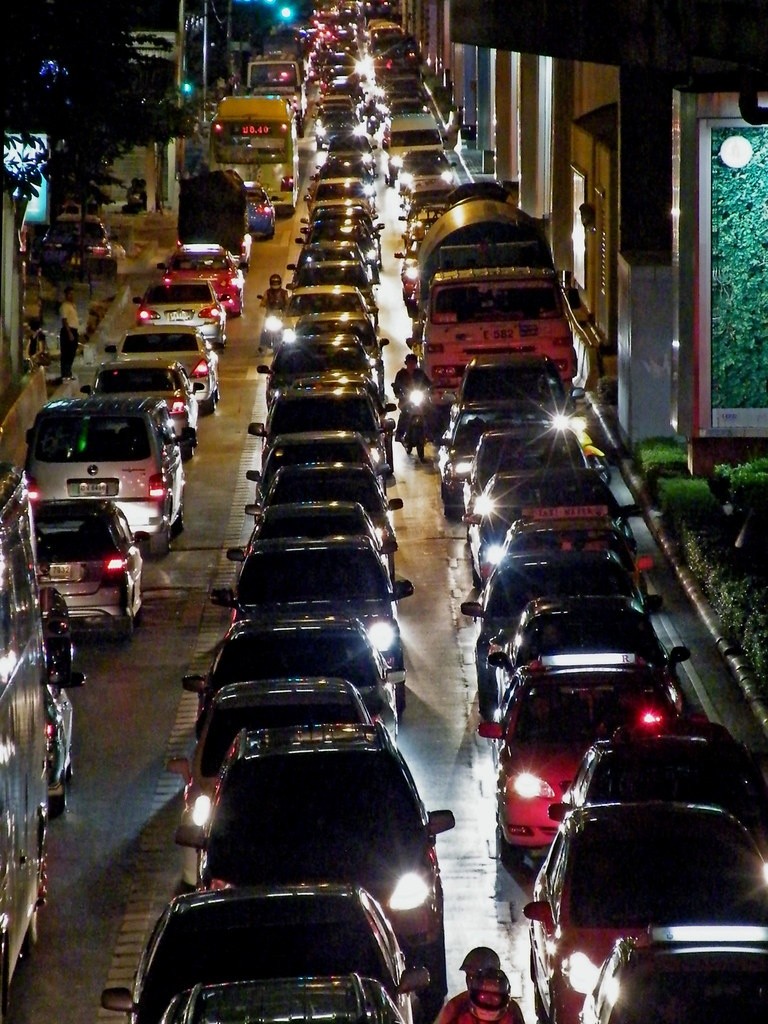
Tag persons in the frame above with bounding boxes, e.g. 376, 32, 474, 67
26, 318, 47, 367
60, 287, 80, 379
122, 178, 147, 214
259, 275, 289, 346
361, 100, 384, 131
433, 947, 525, 1024
393, 354, 434, 442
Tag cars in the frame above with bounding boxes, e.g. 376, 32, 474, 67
548, 710, 768, 864
165, 675, 374, 820
158, 971, 407, 1024
520, 800, 768, 1024
22, 0, 666, 819
474, 651, 710, 867
578, 922, 768, 1024
97, 878, 432, 1024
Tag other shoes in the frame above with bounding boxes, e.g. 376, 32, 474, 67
396, 433, 402, 442
426, 428, 434, 440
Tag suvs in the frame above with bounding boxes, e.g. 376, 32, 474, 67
173, 722, 457, 1002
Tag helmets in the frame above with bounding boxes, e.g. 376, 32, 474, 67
459, 947, 500, 977
270, 274, 281, 290
467, 967, 510, 1021
405, 354, 418, 365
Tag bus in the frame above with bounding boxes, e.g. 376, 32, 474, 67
0, 459, 89, 1024
